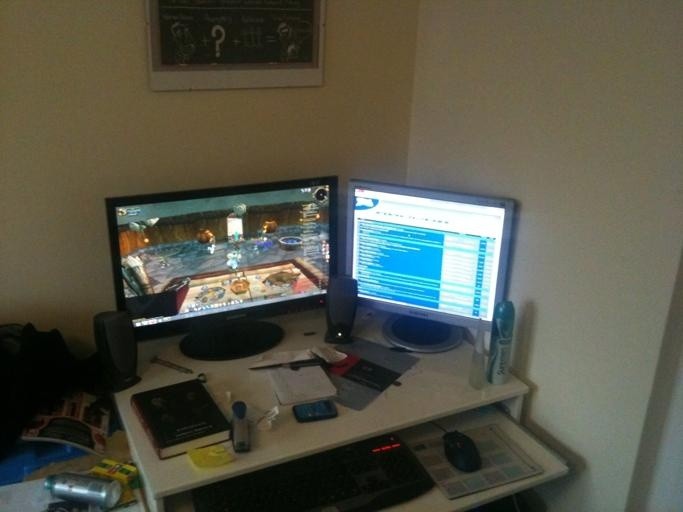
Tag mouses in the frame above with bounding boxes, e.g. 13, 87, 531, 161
442, 430, 482, 472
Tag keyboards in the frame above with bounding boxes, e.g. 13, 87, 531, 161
191, 430, 435, 512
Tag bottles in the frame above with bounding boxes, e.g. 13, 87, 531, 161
487, 300, 515, 385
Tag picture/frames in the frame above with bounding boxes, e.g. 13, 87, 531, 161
147, 0, 325, 91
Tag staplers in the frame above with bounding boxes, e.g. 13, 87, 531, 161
229, 401, 250, 452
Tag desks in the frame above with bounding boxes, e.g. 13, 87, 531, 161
113, 293, 570, 512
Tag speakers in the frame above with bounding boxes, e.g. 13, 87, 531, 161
326, 277, 358, 345
92, 310, 142, 393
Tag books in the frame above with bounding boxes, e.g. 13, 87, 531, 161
130, 377, 234, 462
20, 391, 113, 459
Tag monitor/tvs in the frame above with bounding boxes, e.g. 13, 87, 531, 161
104, 175, 338, 363
344, 179, 517, 354
